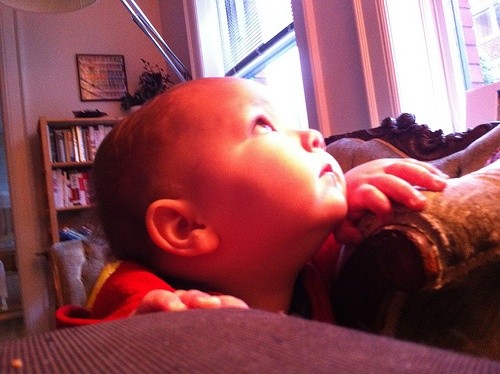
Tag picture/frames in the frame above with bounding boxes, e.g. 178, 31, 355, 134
76, 54, 119, 103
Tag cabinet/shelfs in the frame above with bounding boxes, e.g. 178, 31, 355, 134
37, 116, 123, 308
0, 247, 23, 321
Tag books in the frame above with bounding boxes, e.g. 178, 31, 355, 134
51, 124, 115, 243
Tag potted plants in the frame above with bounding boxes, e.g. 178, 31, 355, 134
119, 58, 175, 112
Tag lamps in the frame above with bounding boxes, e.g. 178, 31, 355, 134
0, 0, 193, 83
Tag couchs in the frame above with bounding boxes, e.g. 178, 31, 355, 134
0, 113, 500, 374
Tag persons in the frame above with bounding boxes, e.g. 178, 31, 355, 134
54, 77, 448, 374
